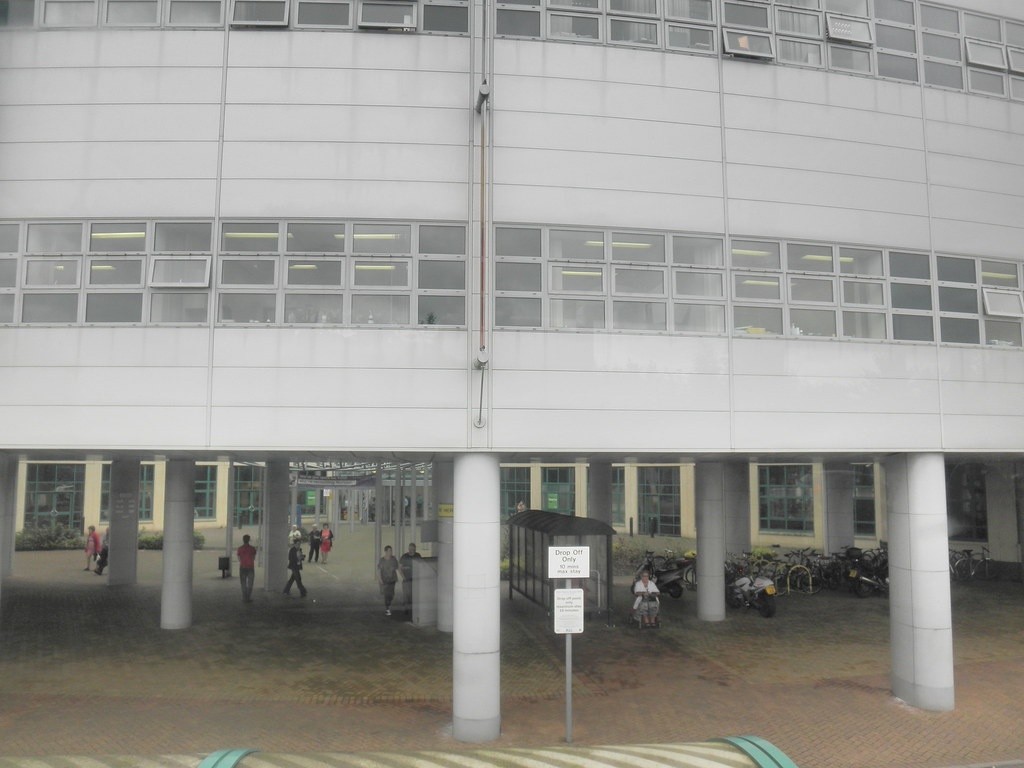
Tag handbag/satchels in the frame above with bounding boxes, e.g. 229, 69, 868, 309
642, 594, 657, 603
94, 553, 101, 563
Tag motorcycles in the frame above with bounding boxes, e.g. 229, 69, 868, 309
729, 569, 775, 618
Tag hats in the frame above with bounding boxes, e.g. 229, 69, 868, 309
312, 524, 317, 526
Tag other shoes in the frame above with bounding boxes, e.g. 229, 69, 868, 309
283, 591, 292, 595
323, 559, 327, 563
388, 606, 392, 611
651, 623, 657, 630
404, 610, 409, 616
300, 593, 306, 598
94, 569, 101, 575
84, 568, 89, 571
643, 622, 651, 629
385, 609, 391, 616
321, 559, 324, 563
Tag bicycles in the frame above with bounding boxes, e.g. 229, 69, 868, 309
949, 545, 998, 581
726, 539, 889, 598
631, 548, 696, 591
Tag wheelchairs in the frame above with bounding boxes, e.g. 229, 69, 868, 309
626, 590, 662, 631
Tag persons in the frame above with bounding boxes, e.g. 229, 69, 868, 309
633, 570, 660, 627
237, 534, 256, 602
83, 525, 109, 576
308, 523, 333, 563
283, 539, 310, 597
289, 525, 301, 546
377, 546, 399, 616
399, 543, 421, 615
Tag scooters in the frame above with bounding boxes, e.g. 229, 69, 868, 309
631, 550, 683, 598
